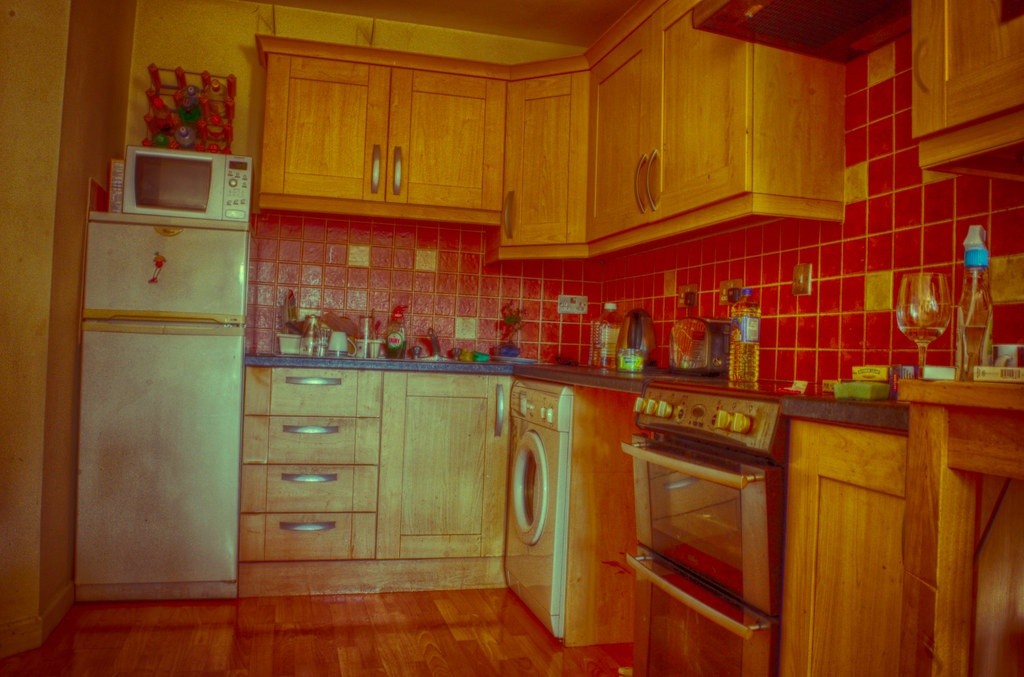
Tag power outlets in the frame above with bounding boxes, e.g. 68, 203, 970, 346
678, 284, 696, 308
719, 279, 742, 305
558, 295, 588, 314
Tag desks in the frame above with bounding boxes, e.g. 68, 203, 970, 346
897, 378, 1024, 677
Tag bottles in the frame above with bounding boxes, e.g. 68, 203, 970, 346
299, 314, 323, 357
729, 288, 760, 383
588, 302, 624, 370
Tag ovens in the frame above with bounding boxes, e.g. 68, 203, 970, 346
615, 434, 783, 676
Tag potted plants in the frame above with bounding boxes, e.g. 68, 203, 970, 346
493, 313, 522, 356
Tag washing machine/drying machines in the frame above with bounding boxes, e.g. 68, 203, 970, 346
504, 378, 573, 638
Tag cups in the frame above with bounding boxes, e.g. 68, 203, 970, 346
358, 318, 373, 360
326, 331, 356, 358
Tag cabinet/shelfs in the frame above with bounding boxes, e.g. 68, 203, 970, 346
777, 418, 1023, 677
244, 365, 511, 589
910, 0, 1024, 182
253, 33, 511, 227
483, 0, 846, 264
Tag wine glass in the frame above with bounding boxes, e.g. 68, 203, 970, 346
896, 272, 952, 378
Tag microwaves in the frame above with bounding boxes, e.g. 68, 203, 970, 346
122, 145, 252, 223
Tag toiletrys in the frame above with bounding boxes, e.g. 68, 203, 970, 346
385, 305, 409, 359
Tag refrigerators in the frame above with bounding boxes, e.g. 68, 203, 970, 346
73, 208, 249, 601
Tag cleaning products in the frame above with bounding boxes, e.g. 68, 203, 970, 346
953, 224, 994, 383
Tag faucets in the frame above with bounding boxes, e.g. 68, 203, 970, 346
411, 327, 463, 363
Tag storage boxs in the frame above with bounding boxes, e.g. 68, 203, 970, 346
276, 333, 387, 359
822, 365, 917, 401
972, 344, 1024, 383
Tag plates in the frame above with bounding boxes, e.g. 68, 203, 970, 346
491, 356, 536, 364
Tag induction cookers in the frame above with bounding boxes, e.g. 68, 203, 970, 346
633, 374, 837, 466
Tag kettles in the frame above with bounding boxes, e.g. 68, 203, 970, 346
616, 308, 656, 372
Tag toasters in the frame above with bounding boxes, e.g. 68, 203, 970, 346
667, 318, 730, 378
